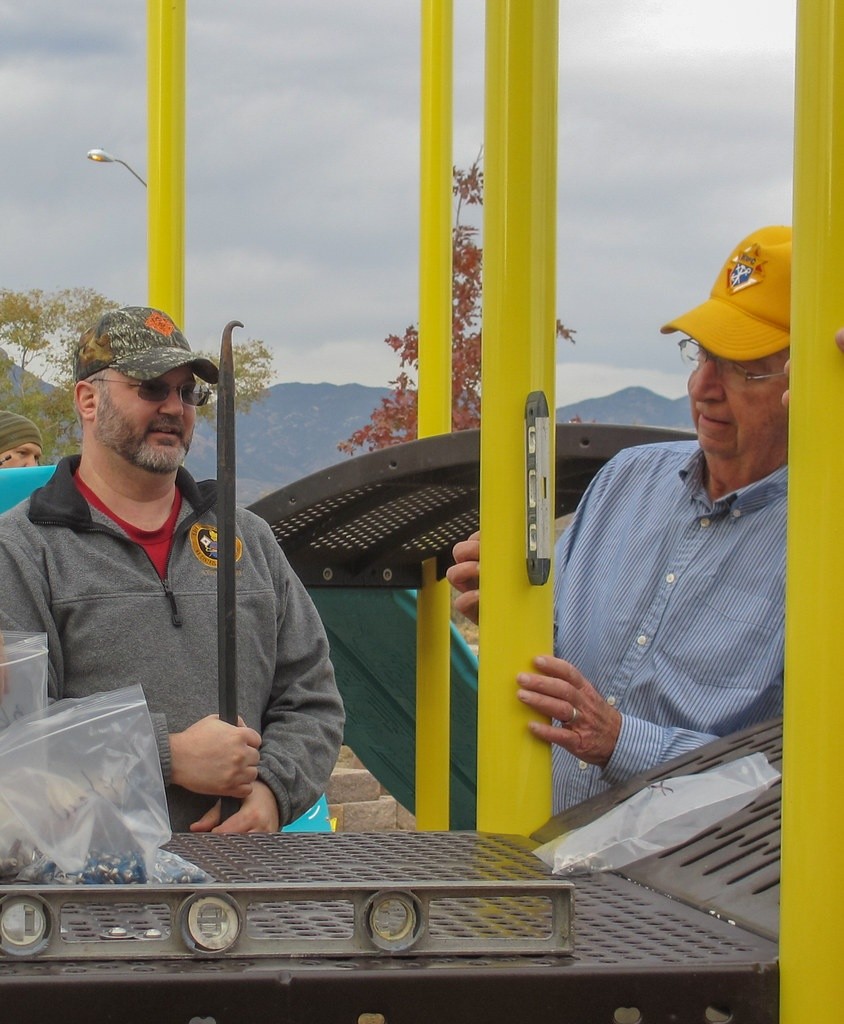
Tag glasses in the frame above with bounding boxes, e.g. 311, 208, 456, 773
89, 379, 212, 406
678, 337, 785, 387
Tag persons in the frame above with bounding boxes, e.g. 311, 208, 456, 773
0, 308, 345, 835
0, 411, 42, 468
446, 224, 796, 814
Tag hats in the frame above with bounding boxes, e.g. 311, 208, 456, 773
0, 410, 42, 454
73, 308, 219, 387
660, 225, 793, 361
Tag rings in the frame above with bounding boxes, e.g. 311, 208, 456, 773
562, 707, 577, 725
